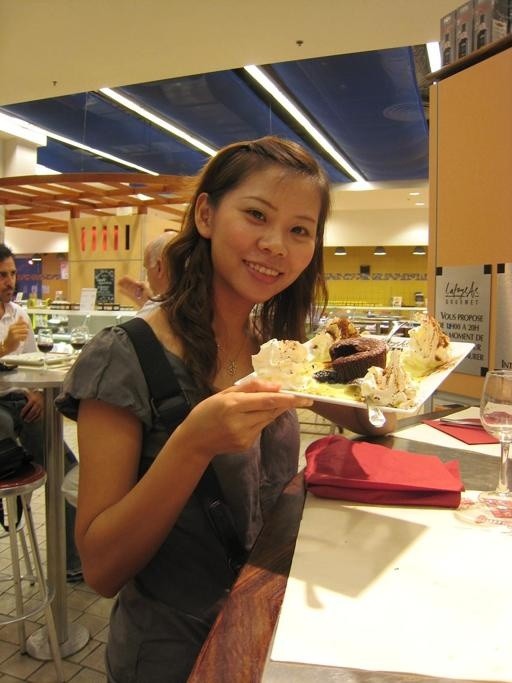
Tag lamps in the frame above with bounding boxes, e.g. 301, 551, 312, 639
333, 247, 427, 255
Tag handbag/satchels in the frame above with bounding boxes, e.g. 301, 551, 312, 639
0, 438, 34, 482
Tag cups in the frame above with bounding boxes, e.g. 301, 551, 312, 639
69, 326, 85, 349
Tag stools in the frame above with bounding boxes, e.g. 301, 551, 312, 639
1, 462, 66, 682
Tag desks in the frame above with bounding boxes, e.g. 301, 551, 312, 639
185, 406, 512, 682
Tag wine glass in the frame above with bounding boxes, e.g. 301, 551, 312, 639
454, 369, 512, 529
37, 328, 54, 375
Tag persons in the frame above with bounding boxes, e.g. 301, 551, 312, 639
51, 135, 399, 681
113, 229, 180, 320
0, 244, 83, 582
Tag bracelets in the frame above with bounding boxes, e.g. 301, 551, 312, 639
1, 338, 7, 355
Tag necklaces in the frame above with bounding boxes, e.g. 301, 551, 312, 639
207, 325, 255, 378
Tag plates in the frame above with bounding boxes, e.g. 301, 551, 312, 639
232, 333, 476, 416
1, 350, 71, 366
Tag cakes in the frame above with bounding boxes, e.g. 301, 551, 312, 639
251, 309, 453, 409
